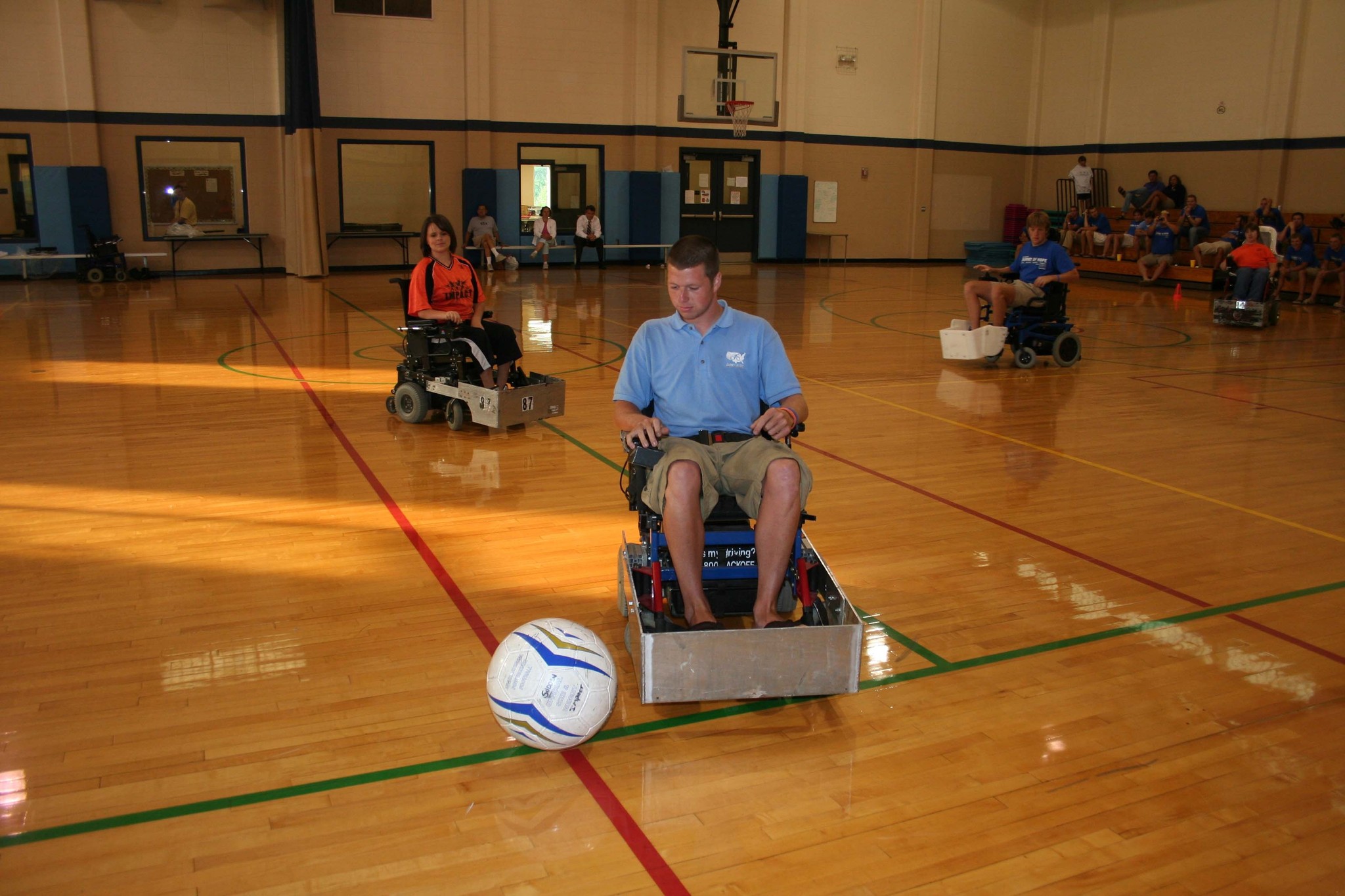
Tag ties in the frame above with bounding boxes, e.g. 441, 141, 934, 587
587, 221, 591, 235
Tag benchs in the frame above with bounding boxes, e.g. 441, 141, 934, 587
463, 243, 674, 270
1015, 206, 1345, 301
0, 251, 168, 284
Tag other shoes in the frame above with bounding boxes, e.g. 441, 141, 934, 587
598, 265, 606, 270
575, 263, 581, 269
1304, 298, 1314, 305
1105, 256, 1114, 259
1334, 302, 1345, 307
1140, 280, 1154, 287
1096, 256, 1105, 259
1275, 293, 1281, 300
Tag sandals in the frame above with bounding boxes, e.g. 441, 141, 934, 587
1116, 215, 1125, 220
1118, 187, 1126, 198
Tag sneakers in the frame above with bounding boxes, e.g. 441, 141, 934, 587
543, 264, 548, 269
495, 254, 506, 262
487, 264, 494, 271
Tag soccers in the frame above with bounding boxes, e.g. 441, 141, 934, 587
486, 616, 617, 751
504, 255, 518, 270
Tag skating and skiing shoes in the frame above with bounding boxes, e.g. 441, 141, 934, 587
530, 253, 536, 259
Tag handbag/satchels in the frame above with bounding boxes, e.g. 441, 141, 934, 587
505, 256, 518, 270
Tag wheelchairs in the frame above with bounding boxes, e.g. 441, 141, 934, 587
76, 225, 132, 284
387, 276, 570, 431
1211, 254, 1281, 330
620, 407, 864, 705
939, 262, 1083, 370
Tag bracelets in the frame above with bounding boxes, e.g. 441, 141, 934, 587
780, 407, 798, 429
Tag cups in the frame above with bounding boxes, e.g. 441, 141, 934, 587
1190, 260, 1196, 268
1116, 254, 1122, 261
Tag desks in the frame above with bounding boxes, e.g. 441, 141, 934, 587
324, 231, 423, 279
161, 232, 271, 278
806, 230, 849, 278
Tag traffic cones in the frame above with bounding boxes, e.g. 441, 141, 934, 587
1172, 283, 1184, 301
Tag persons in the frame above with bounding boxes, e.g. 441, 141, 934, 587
1074, 206, 1112, 258
1176, 194, 1209, 249
963, 209, 1073, 329
1137, 210, 1178, 286
610, 232, 811, 629
530, 206, 557, 270
1129, 211, 1156, 262
1271, 233, 1321, 306
1134, 175, 1188, 216
1193, 215, 1249, 269
407, 214, 522, 392
1252, 197, 1285, 233
1219, 223, 1278, 303
1095, 210, 1144, 260
463, 204, 506, 271
1117, 170, 1166, 220
1277, 212, 1315, 252
573, 205, 607, 270
1059, 205, 1085, 252
1303, 233, 1345, 307
1068, 155, 1094, 213
1015, 227, 1031, 260
171, 185, 198, 229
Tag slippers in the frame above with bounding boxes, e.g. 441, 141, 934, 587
1083, 254, 1095, 258
685, 620, 725, 631
1074, 254, 1083, 257
763, 619, 794, 629
1292, 300, 1303, 304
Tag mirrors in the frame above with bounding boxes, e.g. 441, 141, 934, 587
135, 133, 250, 241
516, 140, 606, 237
0, 132, 39, 245
335, 136, 437, 235
